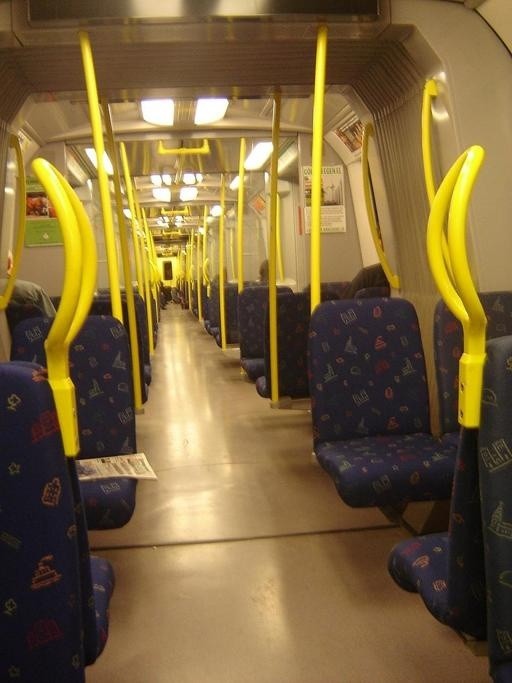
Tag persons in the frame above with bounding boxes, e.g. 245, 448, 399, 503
5, 249, 57, 319
256, 259, 269, 282
